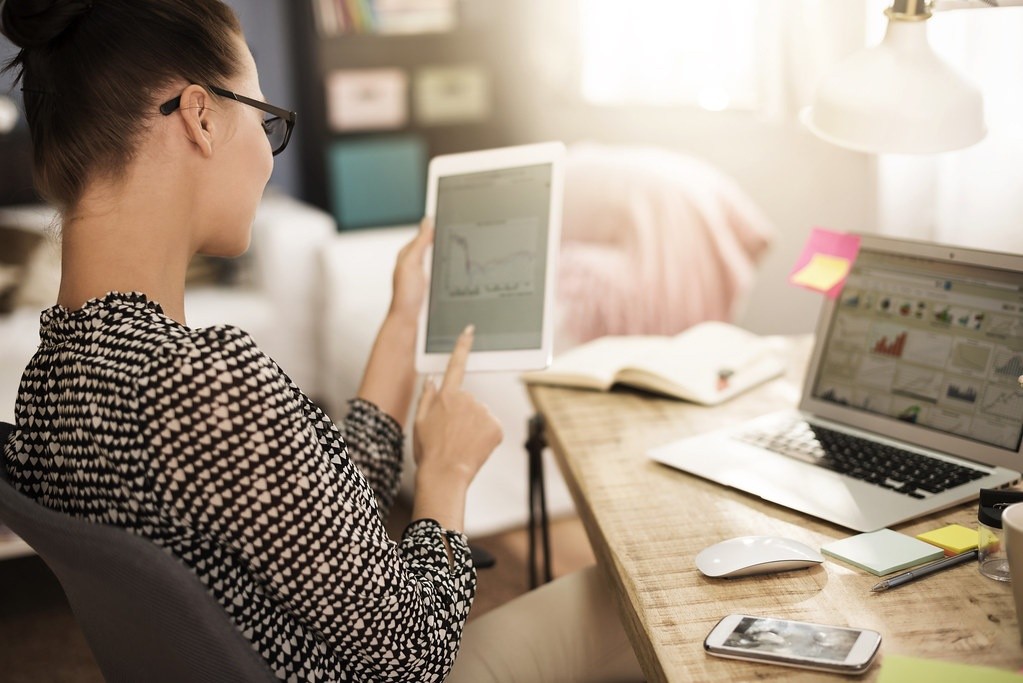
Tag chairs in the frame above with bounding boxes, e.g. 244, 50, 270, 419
0, 419, 286, 683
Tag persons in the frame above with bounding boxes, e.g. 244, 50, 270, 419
1, 0, 648, 683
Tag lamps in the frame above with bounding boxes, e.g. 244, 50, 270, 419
800, 0, 1018, 157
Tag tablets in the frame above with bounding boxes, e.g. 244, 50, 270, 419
414, 141, 566, 373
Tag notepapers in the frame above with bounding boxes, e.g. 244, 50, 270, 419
918, 525, 979, 556
820, 527, 946, 577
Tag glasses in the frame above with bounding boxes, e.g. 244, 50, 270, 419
160, 84, 297, 156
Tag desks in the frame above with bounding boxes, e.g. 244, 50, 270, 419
518, 331, 1023, 683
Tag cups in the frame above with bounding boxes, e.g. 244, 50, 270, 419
978, 504, 1012, 582
1002, 502, 1023, 643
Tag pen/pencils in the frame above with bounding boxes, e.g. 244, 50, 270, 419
868, 549, 979, 592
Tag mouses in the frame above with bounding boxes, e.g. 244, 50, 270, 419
694, 534, 825, 579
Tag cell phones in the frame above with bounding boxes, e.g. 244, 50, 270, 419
703, 613, 882, 675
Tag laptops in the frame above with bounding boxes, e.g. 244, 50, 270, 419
648, 233, 1023, 535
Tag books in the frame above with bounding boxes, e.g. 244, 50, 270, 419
522, 320, 787, 406
314, 0, 490, 130
